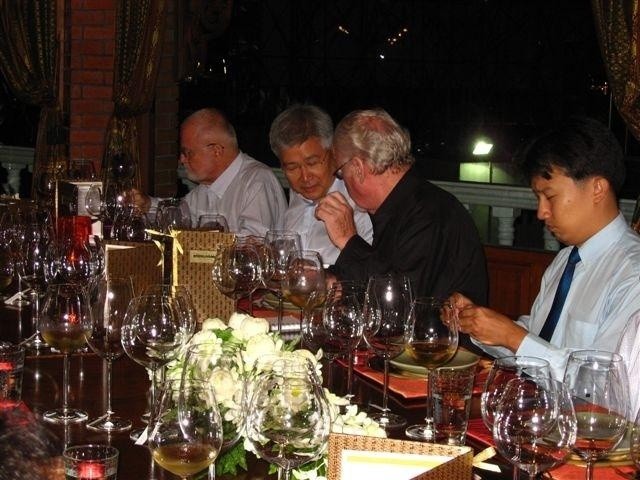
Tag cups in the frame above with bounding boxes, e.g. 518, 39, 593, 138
64, 444, 119, 480
2, 343, 26, 407
426, 366, 476, 448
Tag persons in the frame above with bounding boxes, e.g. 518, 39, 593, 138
603, 310, 640, 427
120, 109, 288, 241
288, 109, 490, 356
441, 116, 638, 430
268, 106, 373, 268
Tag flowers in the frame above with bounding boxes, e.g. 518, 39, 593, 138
148, 306, 387, 478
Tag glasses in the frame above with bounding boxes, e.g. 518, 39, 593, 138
180, 143, 225, 158
333, 159, 353, 180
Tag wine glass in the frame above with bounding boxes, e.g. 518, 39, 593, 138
149, 342, 332, 479
479, 350, 640, 475
209, 228, 460, 441
0, 146, 232, 447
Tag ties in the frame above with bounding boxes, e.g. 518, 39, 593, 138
539, 245, 582, 343
